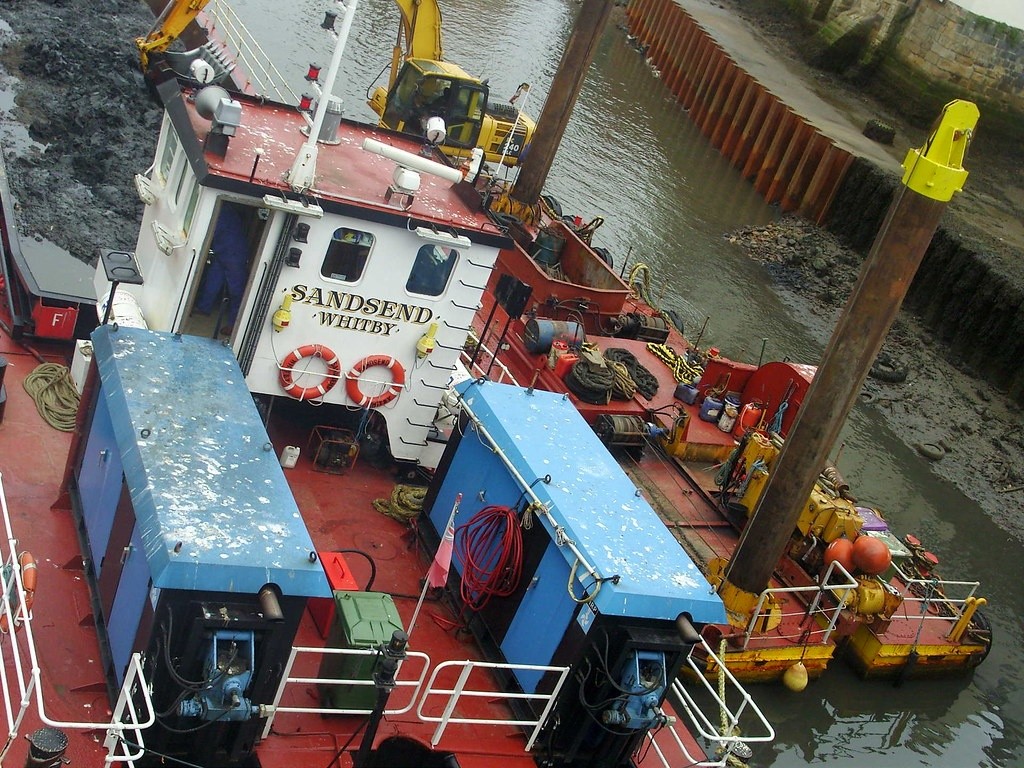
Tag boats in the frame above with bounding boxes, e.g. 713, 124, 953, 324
0, 38, 995, 768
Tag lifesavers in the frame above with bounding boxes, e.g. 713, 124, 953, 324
280, 344, 341, 400
1, 550, 37, 635
345, 355, 406, 409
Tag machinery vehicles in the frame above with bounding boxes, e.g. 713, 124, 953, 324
133, 0, 538, 178
